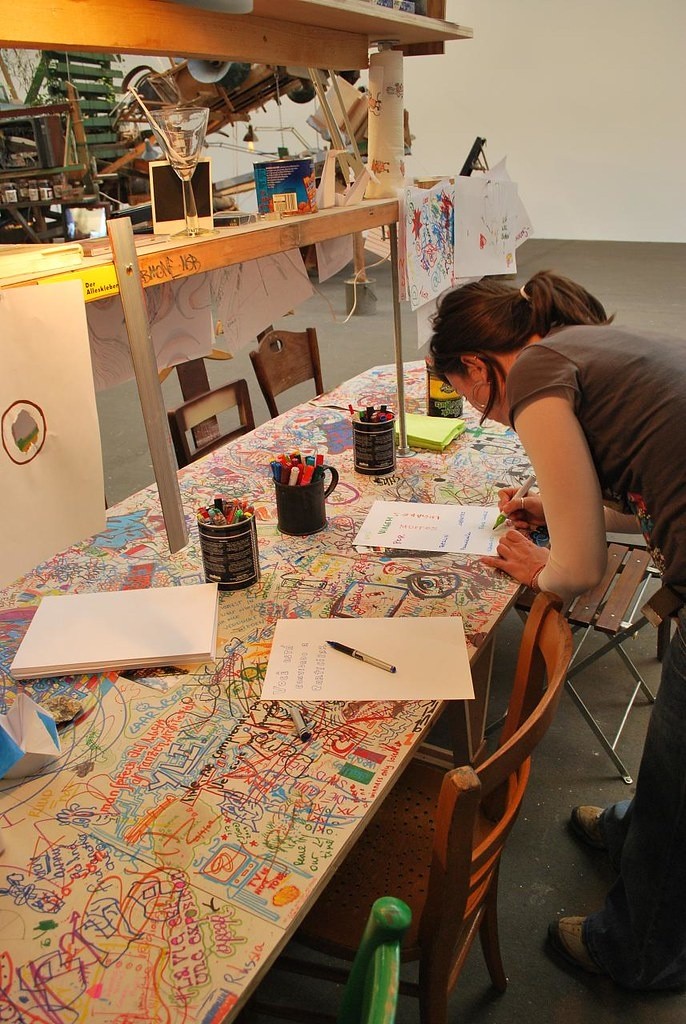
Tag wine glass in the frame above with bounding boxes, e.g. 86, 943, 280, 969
147, 107, 220, 241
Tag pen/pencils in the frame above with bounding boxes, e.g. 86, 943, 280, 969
326, 639, 397, 676
347, 401, 395, 423
423, 355, 432, 370
197, 496, 253, 525
269, 450, 325, 486
492, 472, 536, 530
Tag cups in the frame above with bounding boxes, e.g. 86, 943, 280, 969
425, 354, 462, 417
197, 504, 259, 591
272, 464, 339, 536
352, 409, 396, 475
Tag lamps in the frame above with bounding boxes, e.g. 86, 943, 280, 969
243, 125, 310, 153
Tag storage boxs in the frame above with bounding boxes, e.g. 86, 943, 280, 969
214, 212, 256, 227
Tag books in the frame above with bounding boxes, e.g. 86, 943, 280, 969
9, 582, 219, 680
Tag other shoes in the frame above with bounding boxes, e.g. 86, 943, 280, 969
572, 804, 613, 849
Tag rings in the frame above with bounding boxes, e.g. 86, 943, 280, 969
520, 498, 525, 509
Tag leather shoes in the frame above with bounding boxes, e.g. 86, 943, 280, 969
546, 915, 608, 976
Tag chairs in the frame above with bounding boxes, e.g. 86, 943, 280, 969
232, 896, 413, 1024
486, 540, 683, 785
166, 378, 255, 463
248, 324, 325, 419
237, 590, 573, 1024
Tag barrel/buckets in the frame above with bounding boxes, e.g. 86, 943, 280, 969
254, 157, 318, 217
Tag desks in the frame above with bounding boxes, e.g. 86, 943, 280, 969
0, 359, 550, 1024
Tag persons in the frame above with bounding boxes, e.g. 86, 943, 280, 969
430, 271, 686, 997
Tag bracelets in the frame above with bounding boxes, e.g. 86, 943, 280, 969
531, 563, 546, 594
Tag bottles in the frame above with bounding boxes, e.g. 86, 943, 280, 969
0, 175, 83, 203
